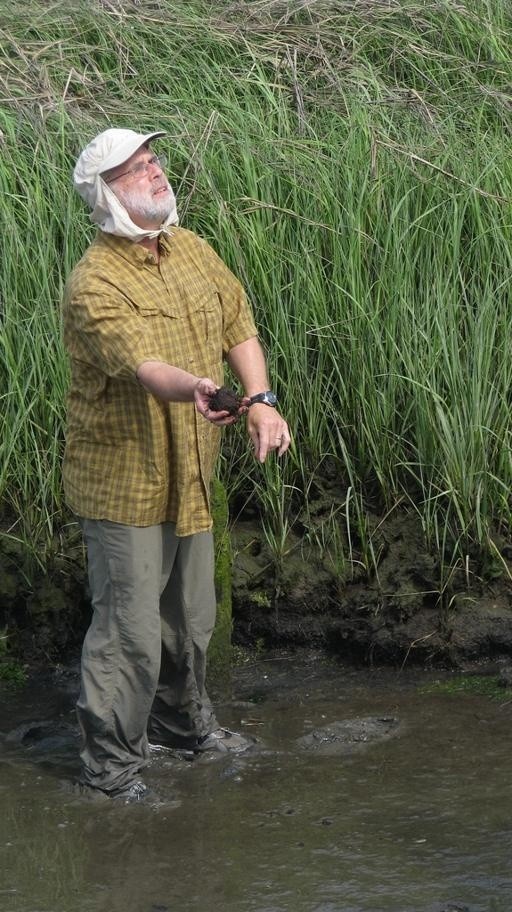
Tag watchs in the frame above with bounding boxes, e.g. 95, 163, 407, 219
246, 390, 280, 408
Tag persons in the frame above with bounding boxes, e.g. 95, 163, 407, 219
60, 125, 294, 806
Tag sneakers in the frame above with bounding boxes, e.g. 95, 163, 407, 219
177, 727, 243, 754
85, 781, 152, 806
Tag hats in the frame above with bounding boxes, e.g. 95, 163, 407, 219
71, 125, 185, 244
